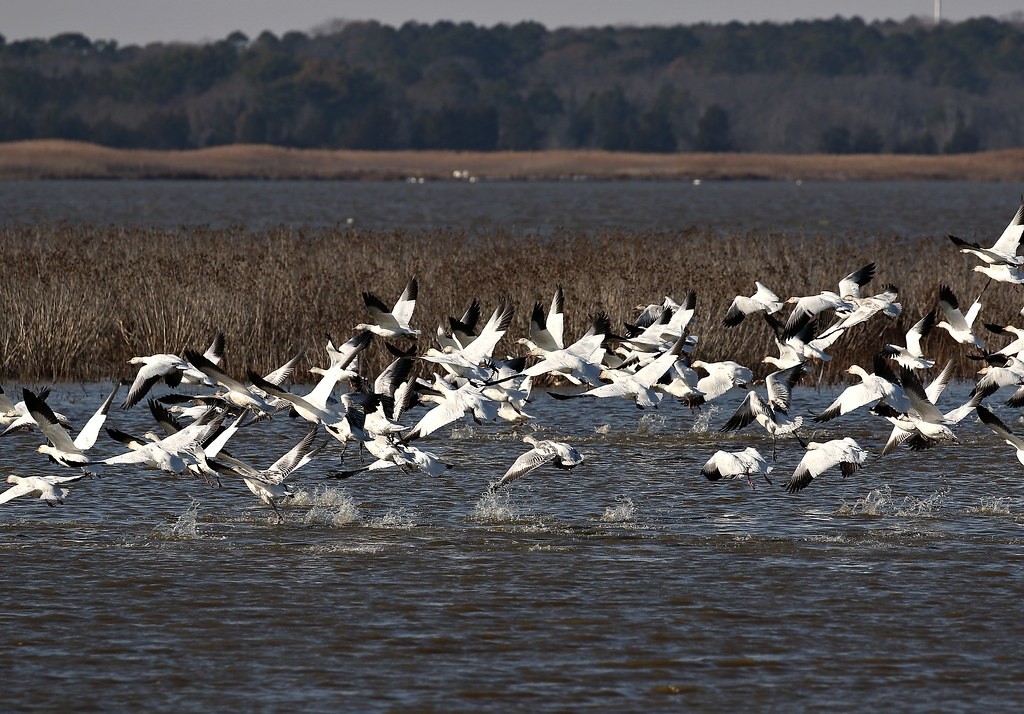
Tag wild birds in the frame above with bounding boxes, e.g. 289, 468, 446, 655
0, 201, 1024, 520
